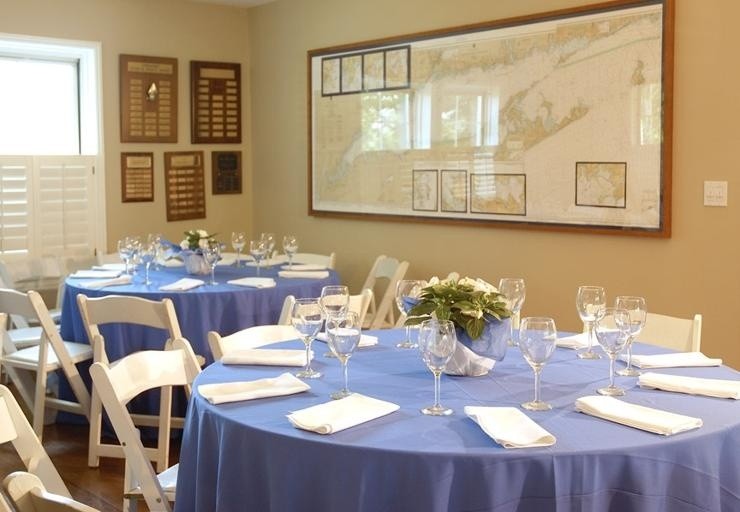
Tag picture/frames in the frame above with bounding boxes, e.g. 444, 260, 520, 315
303, 1, 680, 241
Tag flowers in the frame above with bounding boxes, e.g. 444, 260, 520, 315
180, 230, 225, 250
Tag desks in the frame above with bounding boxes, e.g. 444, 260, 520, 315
173, 325, 740, 511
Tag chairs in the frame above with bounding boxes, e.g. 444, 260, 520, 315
1, 251, 410, 512
581, 311, 702, 354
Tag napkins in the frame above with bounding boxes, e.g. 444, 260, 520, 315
624, 352, 726, 369
556, 333, 600, 351
638, 371, 740, 400
463, 405, 558, 449
575, 395, 702, 437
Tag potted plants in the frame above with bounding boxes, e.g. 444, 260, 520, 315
405, 282, 515, 377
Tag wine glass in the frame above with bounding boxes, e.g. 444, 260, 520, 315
417, 318, 457, 416
519, 316, 557, 411
204, 239, 221, 286
116, 230, 163, 285
498, 276, 527, 346
393, 278, 422, 349
233, 231, 297, 277
577, 286, 647, 397
293, 285, 362, 399
